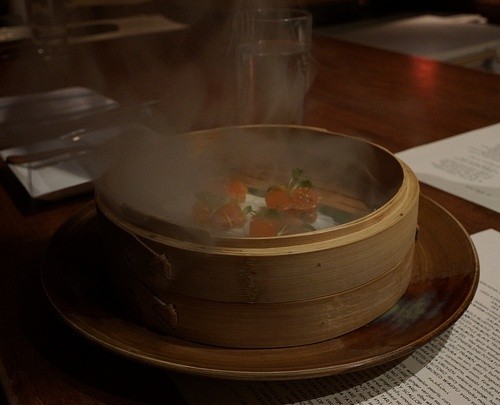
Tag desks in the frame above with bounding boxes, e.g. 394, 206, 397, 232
3, 18, 499, 404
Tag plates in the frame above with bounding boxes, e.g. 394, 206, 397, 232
36, 189, 481, 387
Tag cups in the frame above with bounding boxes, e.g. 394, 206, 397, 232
228, 9, 312, 128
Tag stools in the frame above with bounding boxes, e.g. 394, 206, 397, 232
336, 12, 499, 73
